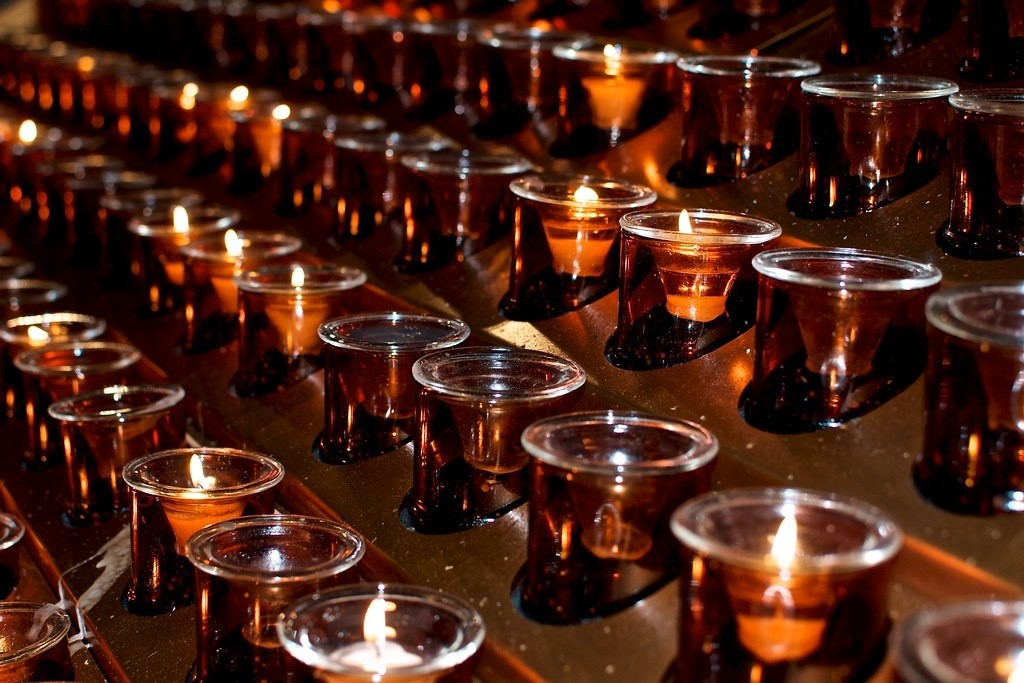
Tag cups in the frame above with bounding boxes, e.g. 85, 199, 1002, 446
0, 0, 1024, 683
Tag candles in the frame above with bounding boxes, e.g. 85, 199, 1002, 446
0, 0, 1024, 683
